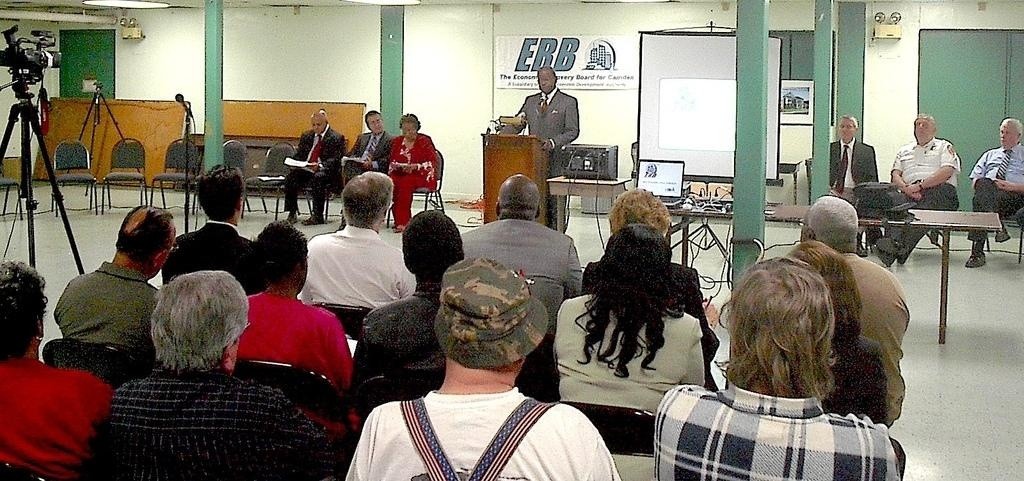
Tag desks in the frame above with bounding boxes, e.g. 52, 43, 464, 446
658, 199, 1001, 347
547, 174, 632, 235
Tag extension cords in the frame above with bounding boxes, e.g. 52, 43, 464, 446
693, 193, 719, 200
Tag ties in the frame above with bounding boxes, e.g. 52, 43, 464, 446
837, 145, 848, 195
309, 135, 322, 163
996, 150, 1011, 181
364, 137, 378, 161
540, 96, 549, 113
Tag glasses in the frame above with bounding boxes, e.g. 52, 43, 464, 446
164, 244, 178, 253
311, 123, 323, 128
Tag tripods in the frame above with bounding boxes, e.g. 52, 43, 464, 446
671, 183, 732, 267
0, 103, 85, 277
62, 81, 147, 196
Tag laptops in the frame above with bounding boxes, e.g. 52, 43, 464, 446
635, 158, 685, 209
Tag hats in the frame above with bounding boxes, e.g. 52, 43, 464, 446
434, 258, 548, 368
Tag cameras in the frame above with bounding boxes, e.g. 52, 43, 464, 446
0, 24, 62, 100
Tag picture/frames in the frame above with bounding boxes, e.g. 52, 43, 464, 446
779, 77, 815, 126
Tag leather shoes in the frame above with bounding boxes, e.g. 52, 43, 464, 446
966, 251, 986, 268
302, 215, 324, 226
287, 214, 297, 224
856, 244, 868, 257
872, 238, 898, 267
995, 229, 1011, 242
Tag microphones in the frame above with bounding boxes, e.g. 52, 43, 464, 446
175, 93, 193, 118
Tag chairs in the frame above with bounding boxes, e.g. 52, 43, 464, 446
223, 138, 250, 215
556, 399, 657, 459
150, 139, 201, 214
355, 370, 442, 425
388, 147, 446, 228
971, 195, 1024, 264
507, 327, 560, 404
309, 300, 372, 344
100, 137, 147, 215
305, 140, 344, 222
232, 357, 352, 443
41, 338, 149, 386
242, 141, 300, 222
0, 158, 24, 220
50, 139, 100, 215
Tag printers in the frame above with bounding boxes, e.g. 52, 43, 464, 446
852, 182, 917, 222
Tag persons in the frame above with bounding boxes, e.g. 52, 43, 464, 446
498, 66, 579, 235
236, 220, 360, 439
830, 114, 882, 258
387, 114, 438, 234
107, 270, 338, 481
296, 171, 417, 310
459, 174, 582, 333
869, 113, 961, 268
282, 111, 346, 226
650, 255, 902, 481
161, 164, 269, 296
550, 224, 706, 481
53, 204, 179, 355
0, 259, 114, 481
341, 110, 390, 177
580, 189, 721, 393
784, 238, 887, 426
344, 256, 625, 481
351, 210, 465, 391
964, 117, 1024, 269
799, 195, 910, 429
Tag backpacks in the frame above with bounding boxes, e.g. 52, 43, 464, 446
852, 182, 917, 242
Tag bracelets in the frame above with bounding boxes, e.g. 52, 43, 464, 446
917, 181, 925, 191
